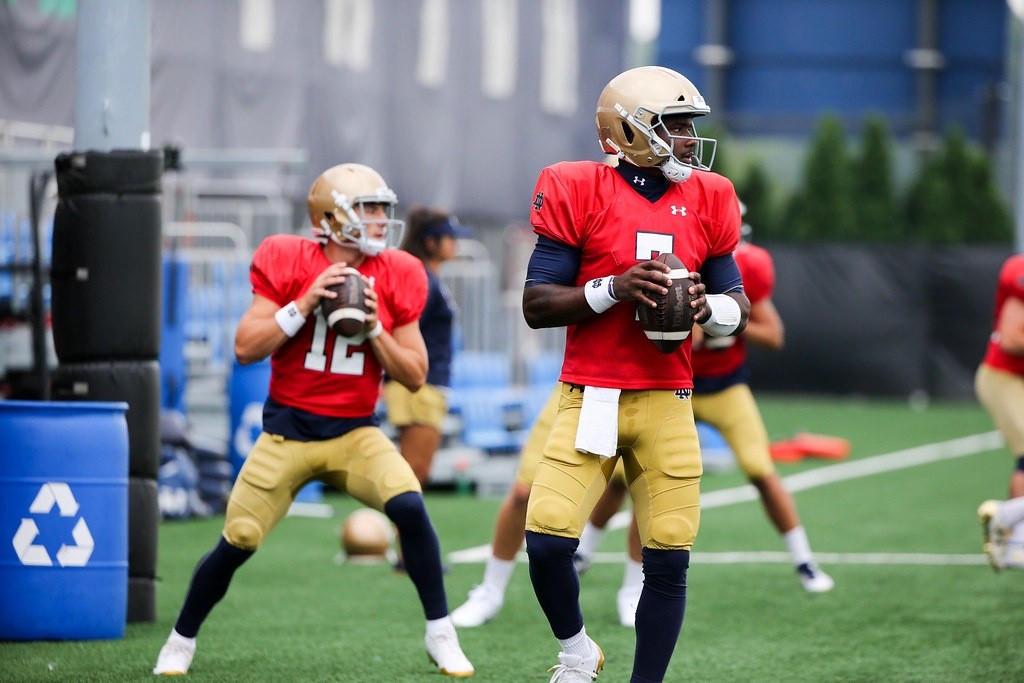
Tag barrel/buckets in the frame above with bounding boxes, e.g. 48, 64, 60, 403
0, 401, 130, 639
230, 364, 318, 507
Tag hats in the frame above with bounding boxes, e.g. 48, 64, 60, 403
423, 212, 472, 238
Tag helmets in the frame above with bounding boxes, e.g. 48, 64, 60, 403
307, 162, 405, 257
596, 66, 717, 184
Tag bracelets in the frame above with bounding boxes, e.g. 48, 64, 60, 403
366, 321, 382, 339
276, 303, 306, 336
584, 275, 620, 312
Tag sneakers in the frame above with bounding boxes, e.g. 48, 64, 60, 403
547, 634, 606, 683
424, 622, 475, 676
452, 582, 502, 627
617, 587, 638, 626
571, 550, 590, 573
155, 632, 197, 674
796, 558, 834, 591
978, 500, 1014, 572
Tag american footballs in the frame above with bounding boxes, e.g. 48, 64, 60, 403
321, 267, 372, 339
637, 252, 698, 353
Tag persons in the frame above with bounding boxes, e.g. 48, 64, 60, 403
450, 385, 647, 624
975, 256, 1024, 498
152, 163, 475, 677
384, 208, 470, 576
573, 241, 833, 590
523, 66, 750, 683
